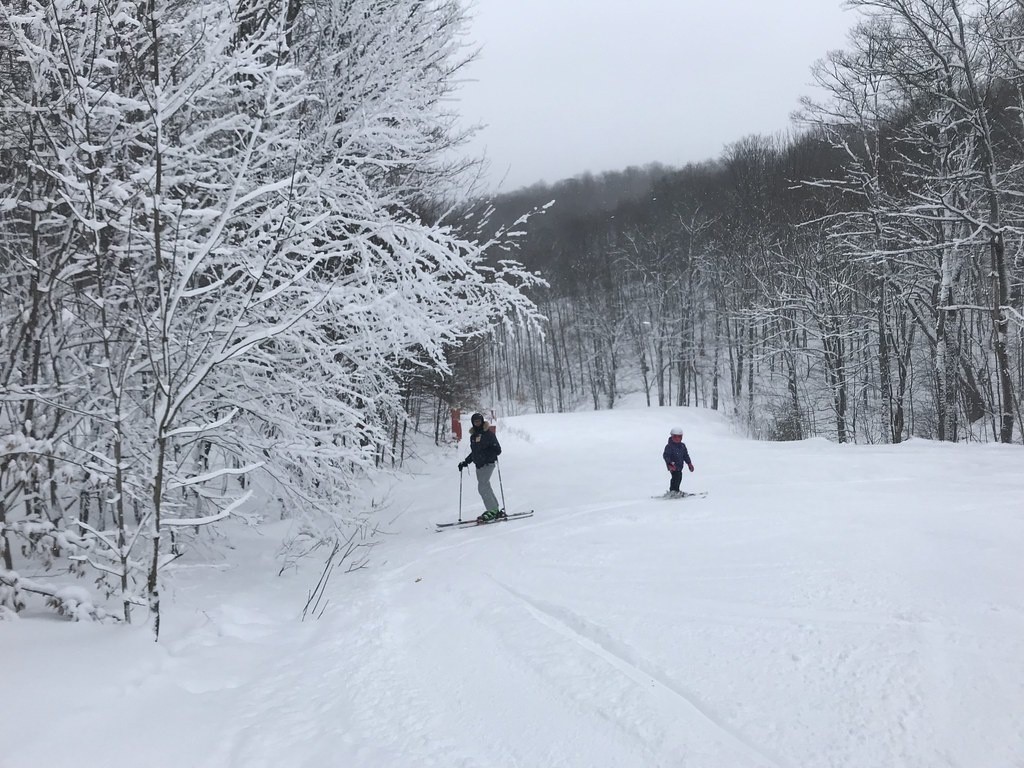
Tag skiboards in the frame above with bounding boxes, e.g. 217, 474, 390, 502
435, 510, 534, 532
651, 491, 708, 500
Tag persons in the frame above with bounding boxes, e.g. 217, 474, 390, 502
663, 428, 694, 496
458, 413, 501, 520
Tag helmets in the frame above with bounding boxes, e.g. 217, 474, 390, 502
472, 413, 484, 429
670, 427, 683, 435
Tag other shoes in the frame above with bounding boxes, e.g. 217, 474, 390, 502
482, 509, 499, 521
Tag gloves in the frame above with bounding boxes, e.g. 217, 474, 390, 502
668, 464, 676, 471
491, 443, 498, 450
688, 463, 694, 472
458, 461, 468, 471
